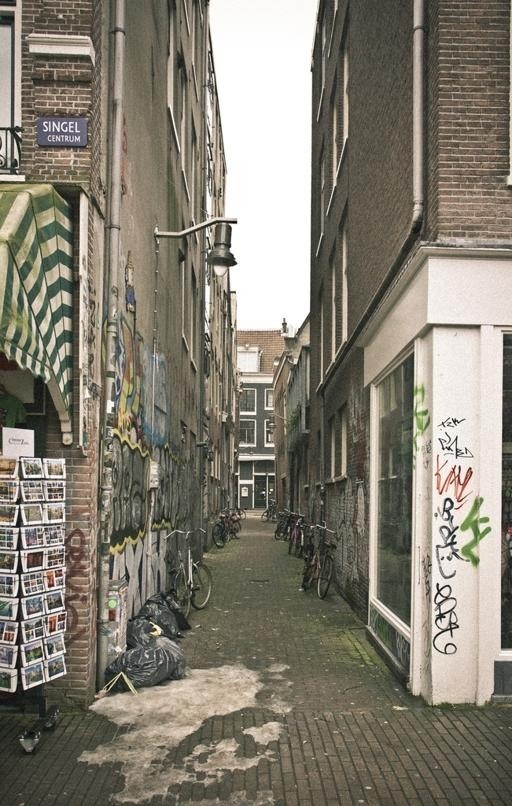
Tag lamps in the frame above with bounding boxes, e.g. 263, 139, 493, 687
154, 217, 237, 277
267, 412, 290, 429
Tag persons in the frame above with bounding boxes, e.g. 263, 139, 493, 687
260, 488, 266, 500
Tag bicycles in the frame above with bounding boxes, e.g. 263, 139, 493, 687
259, 496, 337, 602
208, 490, 249, 548
165, 526, 214, 621
